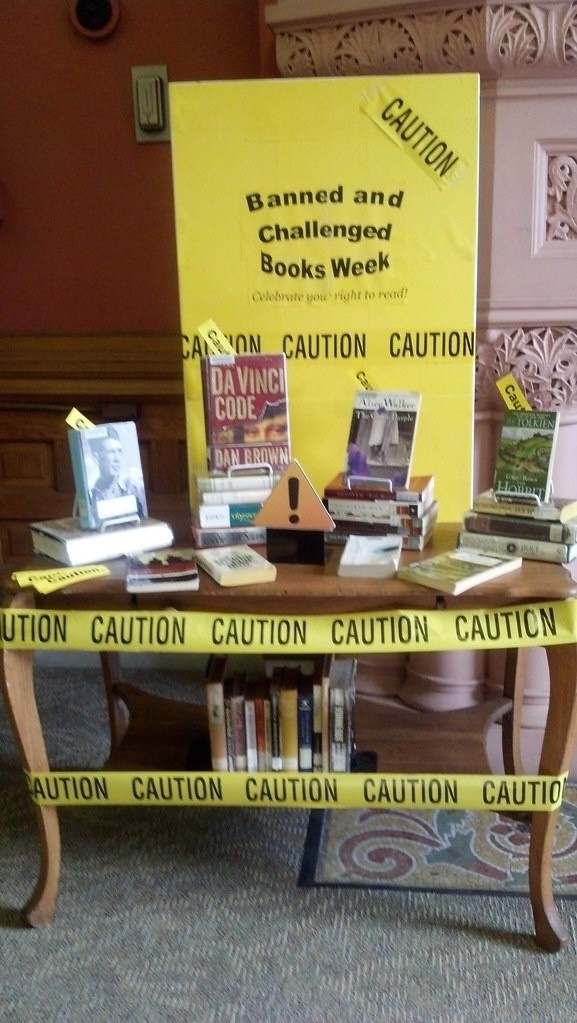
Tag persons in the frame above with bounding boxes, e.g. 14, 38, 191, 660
85, 425, 148, 526
233, 402, 291, 448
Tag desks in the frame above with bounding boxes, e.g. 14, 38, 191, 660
3, 522, 577, 952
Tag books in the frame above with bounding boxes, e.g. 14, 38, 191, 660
199, 353, 291, 476
193, 544, 277, 589
339, 535, 403, 578
25, 421, 174, 567
206, 651, 358, 772
126, 549, 200, 593
321, 389, 438, 550
398, 547, 523, 596
190, 475, 275, 548
456, 410, 576, 564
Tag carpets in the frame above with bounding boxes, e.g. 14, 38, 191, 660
296, 787, 577, 901
